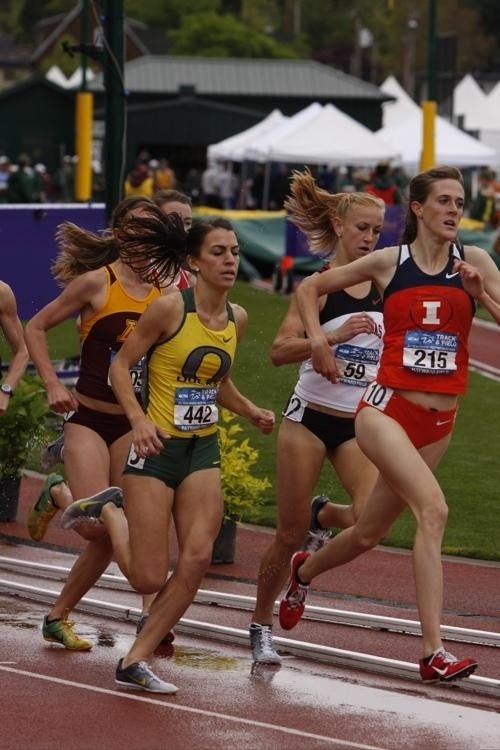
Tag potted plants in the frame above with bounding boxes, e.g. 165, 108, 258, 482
210, 407, 273, 565
0, 373, 53, 522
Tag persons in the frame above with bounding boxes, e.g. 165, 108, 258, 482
1, 149, 412, 208
247, 170, 387, 667
469, 168, 498, 256
21, 194, 181, 653
60, 201, 275, 696
278, 165, 500, 685
0, 281, 30, 419
135, 188, 199, 644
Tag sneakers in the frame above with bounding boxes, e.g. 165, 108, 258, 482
418, 649, 478, 685
42, 608, 93, 650
60, 487, 123, 530
114, 658, 179, 693
249, 622, 281, 665
135, 614, 175, 643
40, 435, 65, 462
27, 472, 65, 541
278, 550, 311, 630
304, 495, 333, 554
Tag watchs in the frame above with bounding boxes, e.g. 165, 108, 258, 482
1, 383, 14, 398
